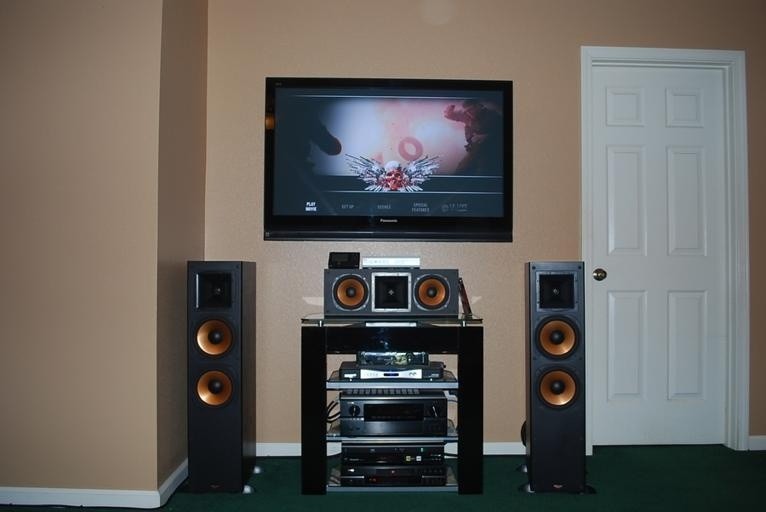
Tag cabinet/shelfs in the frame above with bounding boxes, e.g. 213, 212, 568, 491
300, 313, 486, 496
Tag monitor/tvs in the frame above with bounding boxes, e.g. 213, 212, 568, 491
265, 75, 514, 243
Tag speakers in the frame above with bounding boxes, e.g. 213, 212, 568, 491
186, 260, 256, 491
322, 269, 459, 316
524, 260, 587, 494
